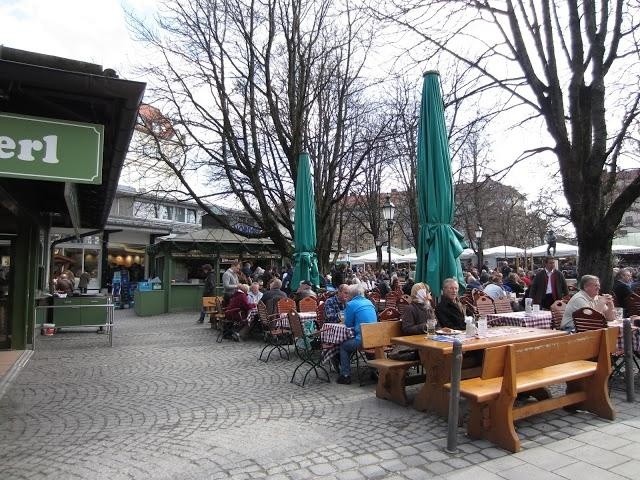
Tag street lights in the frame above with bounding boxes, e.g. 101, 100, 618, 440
474, 224, 484, 281
382, 193, 397, 287
375, 237, 381, 281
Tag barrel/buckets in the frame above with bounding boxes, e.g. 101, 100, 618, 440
43, 323, 56, 336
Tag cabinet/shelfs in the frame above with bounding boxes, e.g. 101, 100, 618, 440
54, 296, 107, 327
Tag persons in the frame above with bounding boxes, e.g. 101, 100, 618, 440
196, 256, 640, 384
51, 261, 144, 298
545, 230, 558, 256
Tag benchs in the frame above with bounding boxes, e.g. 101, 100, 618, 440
443, 326, 622, 454
356, 320, 418, 411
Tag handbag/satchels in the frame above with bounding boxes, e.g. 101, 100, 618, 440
250, 315, 262, 332
390, 345, 418, 361
297, 320, 321, 350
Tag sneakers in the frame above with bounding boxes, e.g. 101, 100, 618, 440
337, 376, 351, 385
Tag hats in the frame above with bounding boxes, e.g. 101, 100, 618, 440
301, 281, 311, 286
202, 264, 212, 271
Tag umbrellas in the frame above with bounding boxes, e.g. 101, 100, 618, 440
411, 69, 469, 306
289, 151, 320, 292
